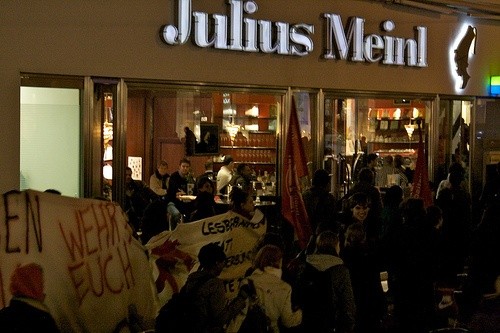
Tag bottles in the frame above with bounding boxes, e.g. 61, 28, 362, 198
219, 132, 276, 162
251, 169, 276, 192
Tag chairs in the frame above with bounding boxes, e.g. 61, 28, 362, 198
167, 202, 183, 231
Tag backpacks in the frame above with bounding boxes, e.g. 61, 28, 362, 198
154, 273, 216, 333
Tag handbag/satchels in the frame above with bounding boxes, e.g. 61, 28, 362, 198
237, 304, 274, 333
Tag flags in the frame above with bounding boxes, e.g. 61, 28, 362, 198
282, 94, 312, 250
411, 142, 433, 209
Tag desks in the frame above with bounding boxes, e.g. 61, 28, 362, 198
256, 185, 277, 197
214, 197, 277, 208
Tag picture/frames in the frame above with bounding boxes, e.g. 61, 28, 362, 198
193, 125, 219, 156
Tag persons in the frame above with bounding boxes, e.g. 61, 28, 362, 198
148, 238, 188, 310
178, 242, 301, 333
282, 154, 500, 333
91, 196, 110, 201
0, 261, 62, 333
125, 157, 255, 244
355, 136, 367, 154
44, 189, 61, 195
184, 127, 196, 157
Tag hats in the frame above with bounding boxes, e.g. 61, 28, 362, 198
198, 243, 226, 267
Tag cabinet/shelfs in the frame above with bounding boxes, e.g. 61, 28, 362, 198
219, 102, 277, 165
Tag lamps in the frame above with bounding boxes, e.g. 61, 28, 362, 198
490, 75, 500, 96
404, 117, 416, 141
